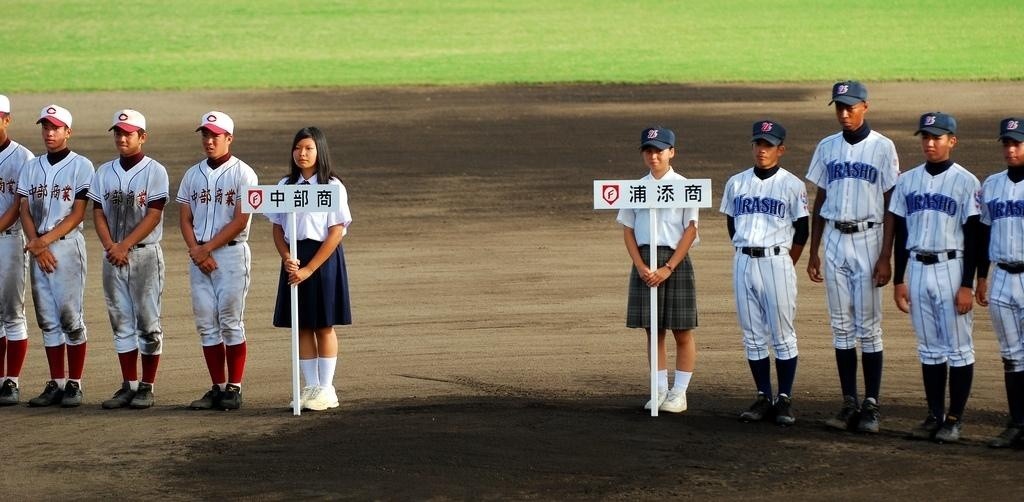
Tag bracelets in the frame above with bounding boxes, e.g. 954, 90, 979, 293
664, 263, 674, 274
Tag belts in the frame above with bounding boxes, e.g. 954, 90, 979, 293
742, 246, 780, 258
997, 261, 1024, 274
835, 221, 874, 234
916, 250, 956, 265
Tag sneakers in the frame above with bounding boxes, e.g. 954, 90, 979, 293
29, 380, 82, 408
644, 388, 688, 413
910, 414, 960, 441
826, 395, 881, 433
102, 382, 154, 409
190, 384, 242, 409
0, 378, 19, 404
990, 422, 1021, 448
290, 385, 339, 411
739, 397, 796, 423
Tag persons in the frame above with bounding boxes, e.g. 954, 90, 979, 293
803, 81, 901, 436
14, 104, 95, 407
84, 108, 170, 410
261, 125, 351, 412
976, 116, 1022, 453
886, 111, 984, 443
174, 109, 259, 410
614, 125, 699, 414
0, 94, 36, 406
719, 120, 810, 425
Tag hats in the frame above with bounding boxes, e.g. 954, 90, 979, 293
36, 104, 72, 129
751, 120, 785, 146
914, 112, 956, 136
638, 128, 675, 150
997, 118, 1024, 142
828, 80, 867, 106
195, 111, 234, 135
108, 109, 146, 133
